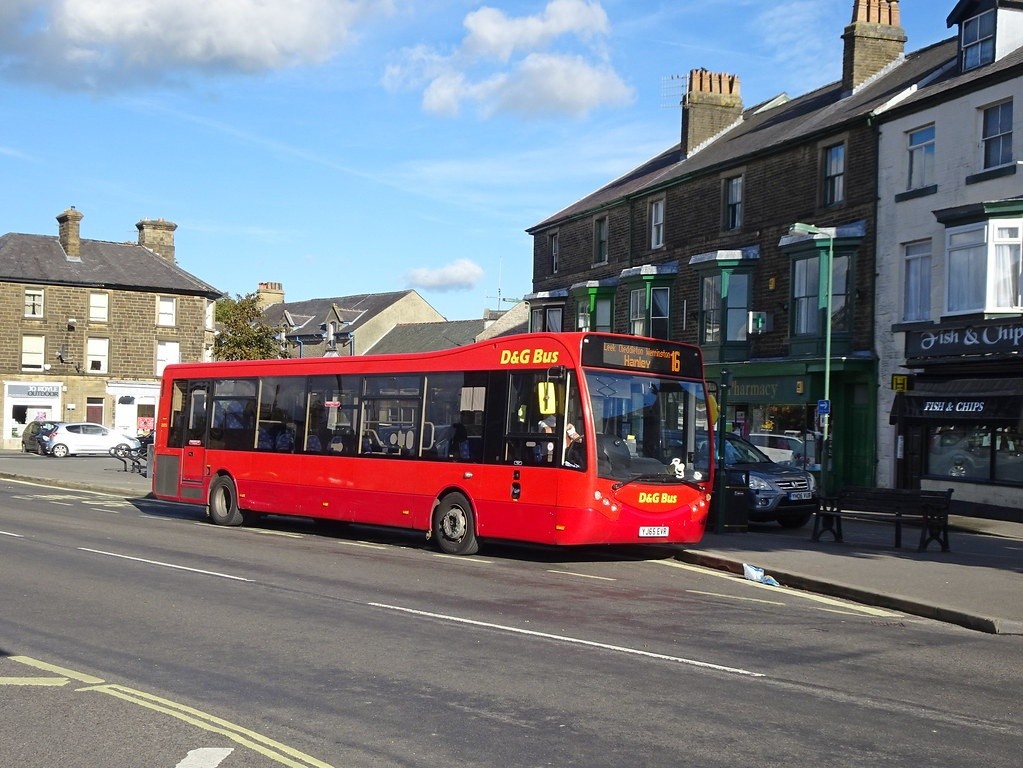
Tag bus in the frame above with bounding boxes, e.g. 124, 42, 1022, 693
152, 332, 720, 557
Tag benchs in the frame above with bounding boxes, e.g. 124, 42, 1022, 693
814, 487, 953, 553
111, 437, 154, 473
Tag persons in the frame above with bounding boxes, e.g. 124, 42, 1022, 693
537, 399, 583, 461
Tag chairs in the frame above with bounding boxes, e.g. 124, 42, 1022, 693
212, 409, 543, 464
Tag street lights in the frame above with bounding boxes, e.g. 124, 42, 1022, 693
787, 223, 835, 510
502, 297, 532, 333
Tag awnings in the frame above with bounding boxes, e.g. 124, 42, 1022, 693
888, 376, 1023, 425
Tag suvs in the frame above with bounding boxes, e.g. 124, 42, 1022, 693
928, 428, 1023, 483
663, 429, 819, 533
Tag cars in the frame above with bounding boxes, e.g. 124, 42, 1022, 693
21, 420, 65, 455
47, 422, 142, 458
137, 430, 153, 456
745, 432, 803, 465
